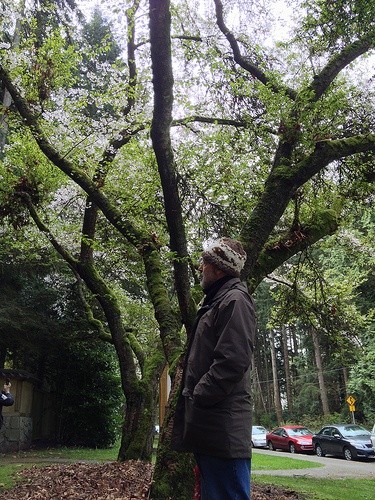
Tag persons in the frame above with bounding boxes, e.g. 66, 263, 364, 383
0, 382, 14, 429
168, 236, 256, 500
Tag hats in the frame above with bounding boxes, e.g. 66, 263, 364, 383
201, 238, 247, 277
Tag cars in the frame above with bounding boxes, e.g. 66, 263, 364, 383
266, 426, 314, 454
251, 426, 269, 448
312, 424, 375, 461
